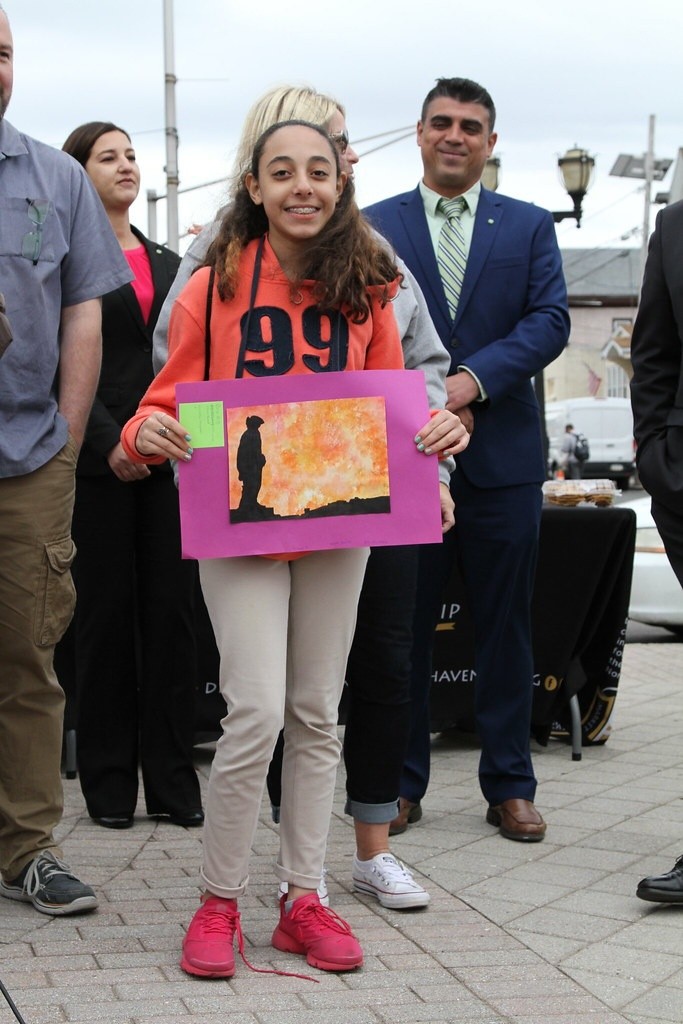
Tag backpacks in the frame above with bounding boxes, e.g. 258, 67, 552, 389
568, 432, 590, 461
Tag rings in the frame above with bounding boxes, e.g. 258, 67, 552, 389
158, 426, 170, 435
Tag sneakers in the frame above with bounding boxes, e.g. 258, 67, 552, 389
0, 848, 99, 914
180, 895, 244, 977
352, 850, 429, 908
272, 893, 363, 971
278, 870, 329, 909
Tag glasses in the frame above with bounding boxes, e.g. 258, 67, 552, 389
329, 129, 349, 153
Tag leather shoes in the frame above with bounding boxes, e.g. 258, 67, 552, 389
386, 797, 422, 835
636, 855, 682, 902
486, 797, 546, 841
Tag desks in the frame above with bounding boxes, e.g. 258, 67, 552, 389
425, 501, 636, 760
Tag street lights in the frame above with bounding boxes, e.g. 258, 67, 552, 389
476, 143, 594, 478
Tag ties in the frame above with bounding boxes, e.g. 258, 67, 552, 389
435, 195, 468, 325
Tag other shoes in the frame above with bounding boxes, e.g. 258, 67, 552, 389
99, 812, 133, 826
168, 812, 203, 825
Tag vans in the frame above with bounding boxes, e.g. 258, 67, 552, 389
543, 396, 638, 489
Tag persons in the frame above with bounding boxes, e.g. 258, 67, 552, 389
152, 85, 430, 906
561, 424, 584, 479
1, 3, 135, 921
63, 121, 204, 828
629, 198, 683, 902
361, 76, 571, 841
122, 121, 470, 986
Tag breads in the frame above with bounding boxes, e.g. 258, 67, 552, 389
548, 484, 614, 507
